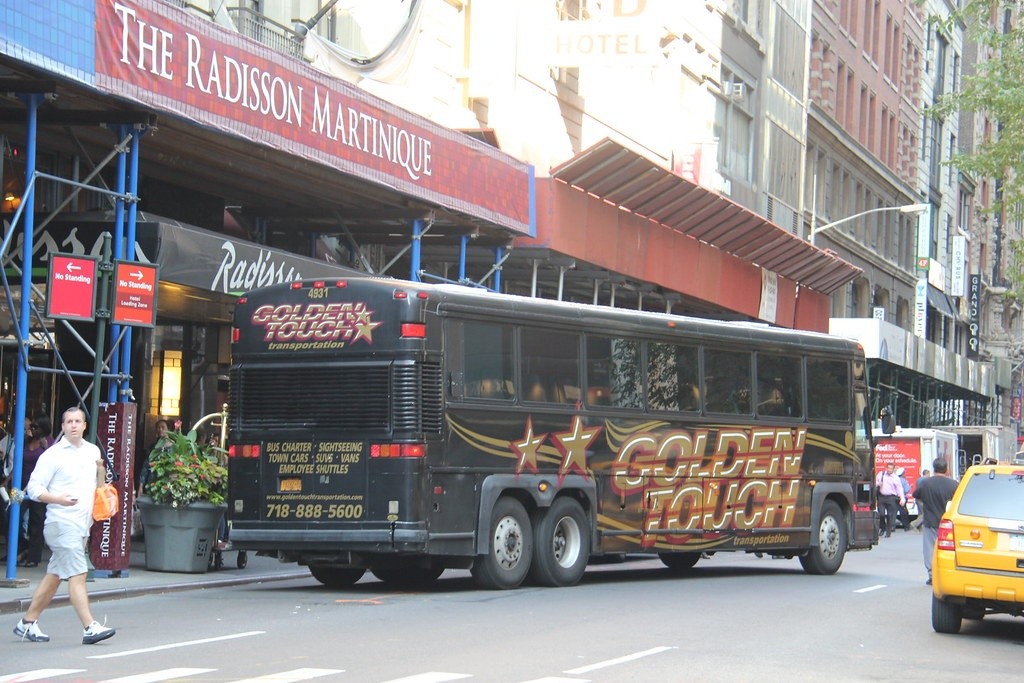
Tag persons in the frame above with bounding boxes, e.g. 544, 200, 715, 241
980, 457, 997, 465
891, 467, 913, 532
910, 470, 930, 532
132, 418, 177, 506
195, 428, 234, 550
876, 463, 906, 537
13, 407, 116, 644
0, 412, 57, 567
912, 457, 959, 585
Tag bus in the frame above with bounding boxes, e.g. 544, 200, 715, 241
217, 274, 900, 591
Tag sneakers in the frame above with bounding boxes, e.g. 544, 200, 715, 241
83, 615, 115, 644
14, 619, 50, 643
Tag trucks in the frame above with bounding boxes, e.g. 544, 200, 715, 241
855, 427, 959, 517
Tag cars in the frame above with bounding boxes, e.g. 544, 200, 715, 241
931, 459, 1024, 634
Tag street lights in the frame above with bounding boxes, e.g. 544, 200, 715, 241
808, 172, 932, 254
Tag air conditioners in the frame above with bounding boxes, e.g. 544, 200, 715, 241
732, 83, 747, 101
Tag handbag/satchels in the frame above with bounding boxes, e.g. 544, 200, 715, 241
92, 483, 119, 521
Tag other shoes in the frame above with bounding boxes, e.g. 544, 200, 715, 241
926, 577, 932, 585
905, 526, 911, 531
879, 529, 885, 536
2, 542, 26, 562
25, 561, 38, 568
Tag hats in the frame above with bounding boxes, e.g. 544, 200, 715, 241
896, 467, 904, 475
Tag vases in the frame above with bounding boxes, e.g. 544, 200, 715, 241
137, 494, 229, 574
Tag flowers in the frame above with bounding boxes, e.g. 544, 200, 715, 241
144, 452, 229, 511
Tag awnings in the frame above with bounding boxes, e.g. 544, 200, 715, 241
549, 136, 864, 296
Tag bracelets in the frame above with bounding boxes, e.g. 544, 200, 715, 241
133, 490, 136, 495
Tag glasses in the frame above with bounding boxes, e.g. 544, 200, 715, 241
29, 424, 39, 429
